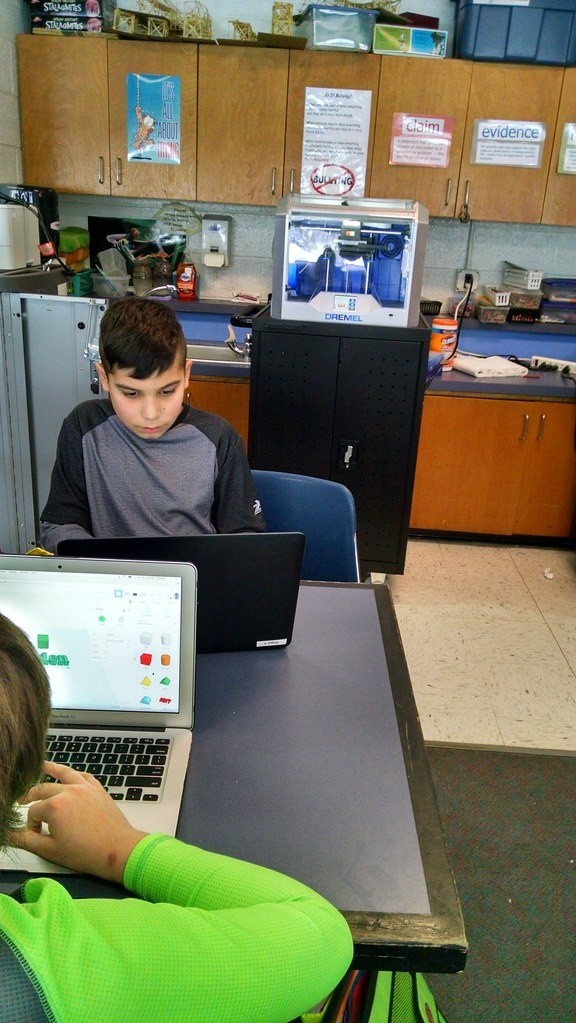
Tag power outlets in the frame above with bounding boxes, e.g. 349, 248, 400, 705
454, 269, 479, 291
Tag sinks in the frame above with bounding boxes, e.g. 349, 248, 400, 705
186, 344, 251, 369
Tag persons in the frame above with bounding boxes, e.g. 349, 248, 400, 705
0, 613, 354, 1023
39, 297, 267, 559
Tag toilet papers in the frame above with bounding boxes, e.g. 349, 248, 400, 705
203, 253, 225, 267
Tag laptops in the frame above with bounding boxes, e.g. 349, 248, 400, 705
0, 552, 198, 879
56, 533, 303, 652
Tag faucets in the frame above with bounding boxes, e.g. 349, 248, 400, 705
141, 284, 179, 298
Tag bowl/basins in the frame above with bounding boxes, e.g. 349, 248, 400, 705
90, 272, 131, 298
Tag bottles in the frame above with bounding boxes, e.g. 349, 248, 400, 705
176, 259, 197, 302
427, 318, 458, 371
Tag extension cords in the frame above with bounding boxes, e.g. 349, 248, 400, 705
530, 355, 576, 374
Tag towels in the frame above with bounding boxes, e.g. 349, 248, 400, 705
452, 355, 529, 378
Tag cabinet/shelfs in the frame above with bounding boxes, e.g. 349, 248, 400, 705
408, 390, 576, 537
250, 301, 433, 576
15, 33, 576, 228
182, 377, 251, 456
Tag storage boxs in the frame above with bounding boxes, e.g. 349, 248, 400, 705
292, 4, 379, 53
452, 0, 576, 66
66, 268, 96, 297
421, 269, 576, 324
28, 0, 104, 35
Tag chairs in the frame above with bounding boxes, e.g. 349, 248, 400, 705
246, 470, 361, 582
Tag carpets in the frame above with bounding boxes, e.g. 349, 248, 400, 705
380, 741, 574, 1023
